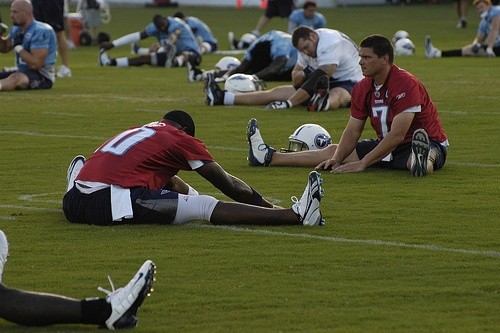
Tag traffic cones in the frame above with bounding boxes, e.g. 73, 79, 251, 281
260, 0, 268, 10
235, 0, 243, 10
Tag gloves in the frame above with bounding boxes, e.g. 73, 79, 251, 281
13, 33, 24, 52
471, 43, 479, 53
486, 47, 496, 57
266, 100, 289, 110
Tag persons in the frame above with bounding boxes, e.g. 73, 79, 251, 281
0, 230, 156, 330
0, 0, 57, 91
229, 1, 327, 50
63, 110, 325, 227
99, 15, 201, 66
425, 0, 500, 57
247, 35, 449, 177
186, 31, 298, 82
255, 0, 295, 31
132, 12, 218, 54
204, 26, 365, 111
31, 0, 71, 77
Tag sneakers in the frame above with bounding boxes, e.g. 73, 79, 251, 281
411, 129, 430, 177
291, 170, 325, 226
245, 118, 276, 166
98, 260, 156, 330
165, 45, 179, 67
425, 35, 438, 58
307, 75, 330, 112
187, 61, 202, 82
56, 65, 72, 78
229, 32, 238, 49
205, 72, 227, 105
131, 41, 140, 54
98, 47, 112, 66
66, 155, 86, 192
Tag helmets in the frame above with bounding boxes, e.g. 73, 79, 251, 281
394, 38, 415, 55
224, 74, 267, 93
215, 56, 240, 71
392, 30, 410, 45
239, 33, 257, 50
288, 124, 332, 152
